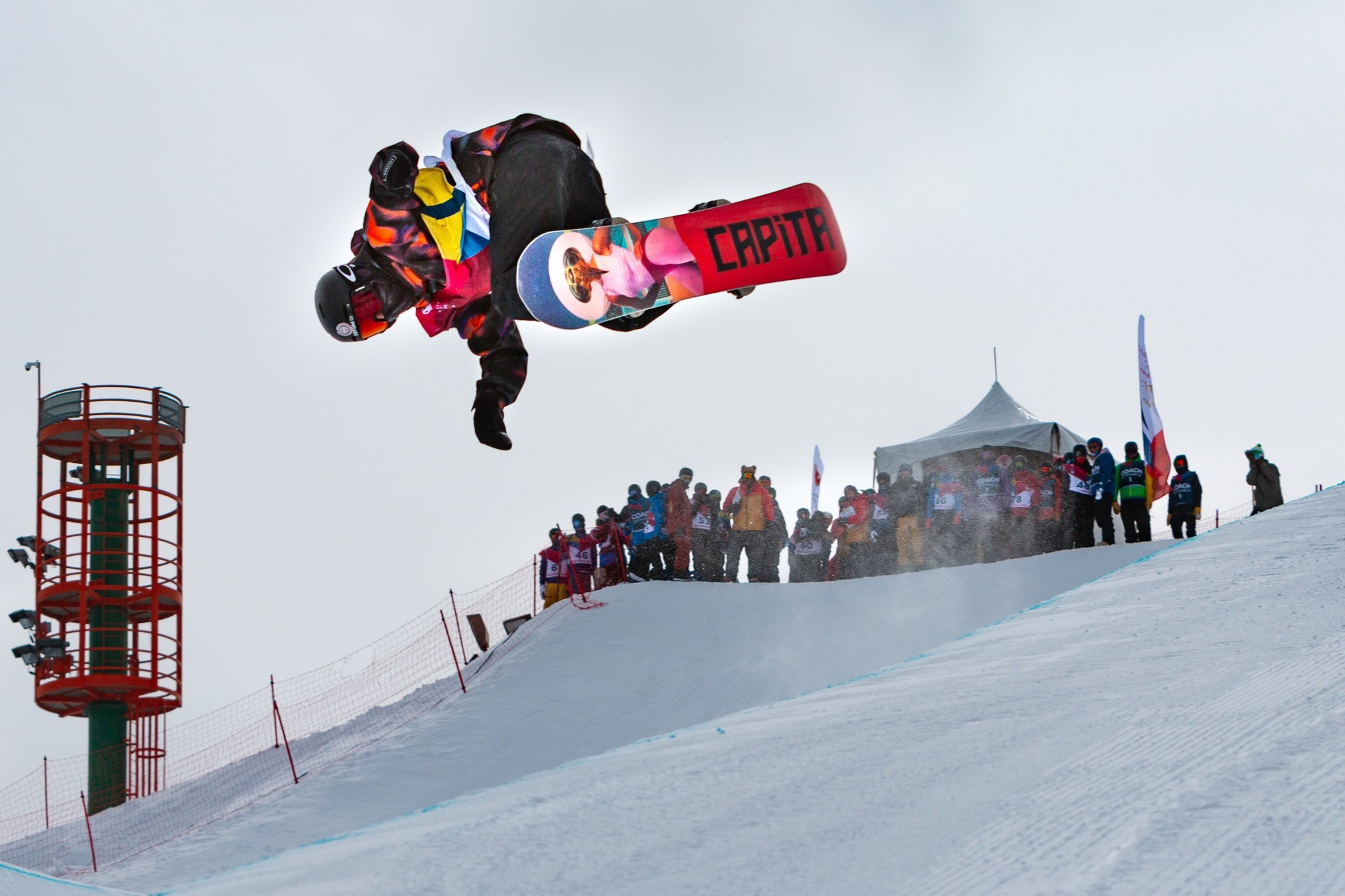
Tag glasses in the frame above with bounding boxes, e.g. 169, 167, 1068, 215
1088, 444, 1099, 451
743, 473, 753, 479
1075, 450, 1087, 456
1014, 461, 1026, 468
770, 493, 775, 498
573, 520, 583, 528
1039, 466, 1052, 473
681, 475, 692, 483
629, 489, 639, 497
599, 511, 608, 519
551, 533, 560, 541
982, 450, 994, 458
759, 480, 770, 487
1066, 458, 1077, 464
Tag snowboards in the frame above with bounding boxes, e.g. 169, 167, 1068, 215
515, 182, 847, 331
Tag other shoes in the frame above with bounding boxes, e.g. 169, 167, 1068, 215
1097, 541, 1109, 546
593, 219, 647, 321
696, 199, 757, 298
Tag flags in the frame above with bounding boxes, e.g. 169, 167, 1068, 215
1138, 316, 1171, 509
811, 445, 824, 515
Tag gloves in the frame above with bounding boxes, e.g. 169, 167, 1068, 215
474, 398, 512, 451
1113, 501, 1122, 514
378, 149, 415, 198
1167, 507, 1201, 526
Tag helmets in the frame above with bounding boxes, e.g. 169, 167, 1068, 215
798, 485, 875, 521
1039, 461, 1053, 469
1012, 455, 1027, 465
315, 262, 415, 342
980, 444, 993, 452
876, 462, 913, 484
549, 466, 778, 543
1053, 437, 1102, 467
1125, 442, 1138, 452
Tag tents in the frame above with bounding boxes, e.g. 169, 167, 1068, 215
872, 381, 1126, 545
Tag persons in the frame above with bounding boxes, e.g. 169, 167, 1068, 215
1113, 442, 1152, 542
709, 490, 730, 582
724, 466, 775, 583
1245, 444, 1283, 513
1167, 455, 1202, 539
316, 112, 755, 450
590, 506, 633, 585
831, 444, 1073, 580
788, 508, 835, 582
689, 482, 717, 582
1054, 443, 1095, 548
559, 514, 597, 597
605, 482, 677, 583
1087, 438, 1116, 546
759, 476, 788, 583
666, 468, 693, 581
538, 528, 569, 610
